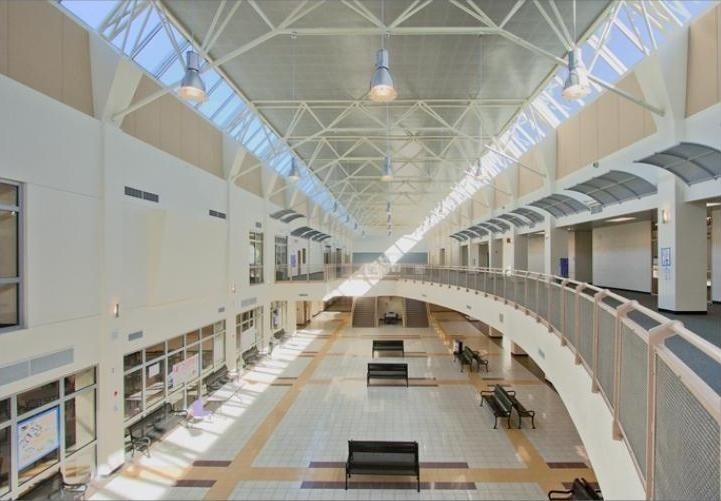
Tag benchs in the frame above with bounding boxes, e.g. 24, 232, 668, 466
242, 346, 263, 368
274, 327, 287, 339
128, 403, 189, 457
453, 346, 474, 372
548, 477, 595, 501
367, 364, 408, 386
466, 346, 488, 372
480, 384, 513, 429
345, 441, 420, 492
205, 364, 234, 396
581, 478, 603, 501
372, 341, 404, 358
499, 384, 535, 429
12, 469, 87, 501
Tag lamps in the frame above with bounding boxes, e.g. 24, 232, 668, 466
379, 30, 396, 183
476, 31, 486, 181
561, 0, 592, 99
368, 0, 398, 102
179, 6, 207, 99
287, 34, 300, 181
